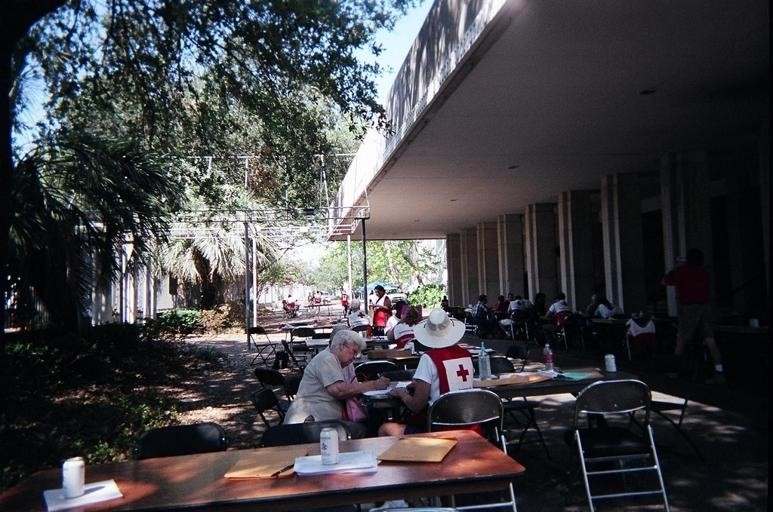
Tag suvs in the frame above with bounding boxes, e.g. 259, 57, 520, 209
369, 287, 407, 306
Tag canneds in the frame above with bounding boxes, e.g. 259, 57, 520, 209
319, 427, 341, 464
604, 353, 617, 372
62, 456, 86, 498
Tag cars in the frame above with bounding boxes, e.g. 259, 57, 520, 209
320, 291, 331, 302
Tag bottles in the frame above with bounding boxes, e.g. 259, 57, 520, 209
478, 342, 492, 380
543, 344, 554, 371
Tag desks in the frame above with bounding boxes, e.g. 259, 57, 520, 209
0, 430, 529, 511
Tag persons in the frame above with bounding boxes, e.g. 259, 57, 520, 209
661, 239, 726, 385
473, 292, 614, 347
441, 296, 448, 312
377, 308, 480, 438
368, 285, 424, 351
340, 291, 361, 316
282, 294, 298, 318
282, 330, 365, 446
324, 325, 380, 438
308, 290, 322, 315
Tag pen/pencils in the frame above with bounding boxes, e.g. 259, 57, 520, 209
271, 453, 308, 477
377, 373, 389, 386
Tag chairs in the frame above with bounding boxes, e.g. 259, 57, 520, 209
136, 422, 228, 459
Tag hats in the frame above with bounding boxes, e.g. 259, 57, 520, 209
414, 308, 466, 349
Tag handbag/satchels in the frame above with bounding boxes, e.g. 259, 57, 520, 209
346, 395, 369, 422
